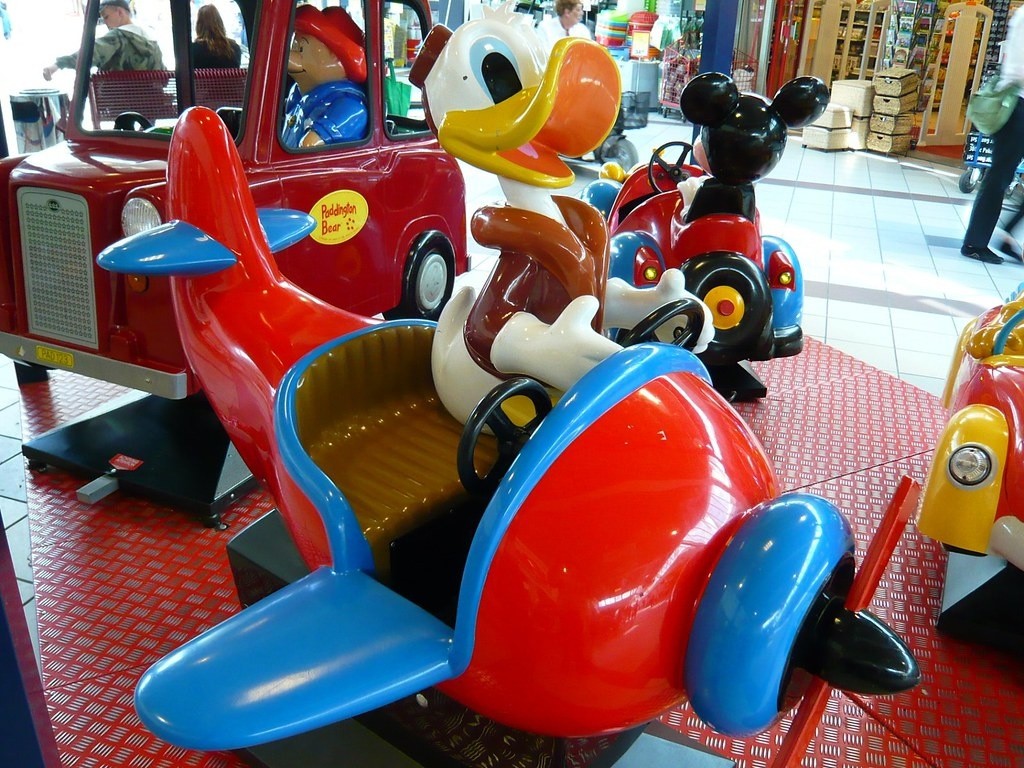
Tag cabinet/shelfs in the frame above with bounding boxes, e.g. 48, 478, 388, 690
825, 1, 891, 91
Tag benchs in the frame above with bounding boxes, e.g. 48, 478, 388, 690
85, 69, 246, 131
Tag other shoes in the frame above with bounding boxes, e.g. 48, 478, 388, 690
960, 245, 1005, 264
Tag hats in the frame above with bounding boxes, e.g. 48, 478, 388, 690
100, 0, 130, 13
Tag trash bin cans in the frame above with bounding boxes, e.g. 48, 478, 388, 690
10, 88, 70, 155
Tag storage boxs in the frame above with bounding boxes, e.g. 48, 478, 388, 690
803, 69, 918, 158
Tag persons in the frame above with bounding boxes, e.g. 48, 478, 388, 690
192, 4, 241, 71
41, 1, 170, 132
537, 0, 591, 62
959, 1, 1024, 264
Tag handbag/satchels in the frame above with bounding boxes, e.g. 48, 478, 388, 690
385, 59, 412, 118
965, 75, 1024, 135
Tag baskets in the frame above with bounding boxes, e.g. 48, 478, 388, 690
619, 91, 651, 129
